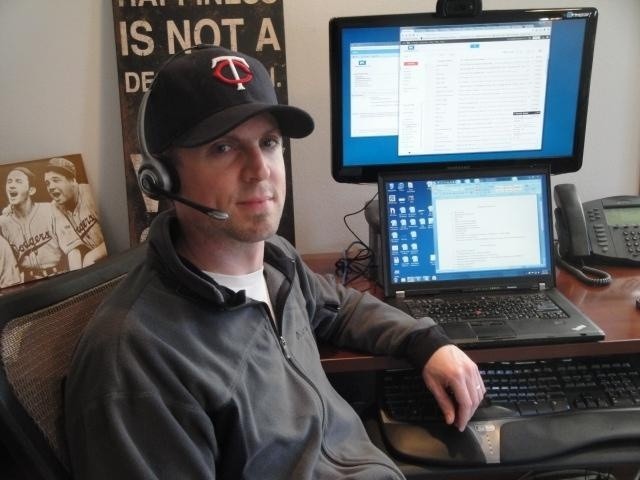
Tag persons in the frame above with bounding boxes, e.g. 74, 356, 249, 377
40, 157, 109, 269
0, 167, 85, 286
53, 41, 490, 480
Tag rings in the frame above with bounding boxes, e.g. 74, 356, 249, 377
476, 385, 481, 390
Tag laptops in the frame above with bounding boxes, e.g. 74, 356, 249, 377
376, 164, 606, 348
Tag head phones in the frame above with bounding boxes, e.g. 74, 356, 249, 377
135, 43, 220, 199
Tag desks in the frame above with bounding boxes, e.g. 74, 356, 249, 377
300, 252, 640, 376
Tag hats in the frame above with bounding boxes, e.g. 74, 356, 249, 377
138, 46, 316, 154
13, 166, 36, 181
44, 157, 77, 177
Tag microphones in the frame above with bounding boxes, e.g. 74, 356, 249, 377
154, 185, 228, 221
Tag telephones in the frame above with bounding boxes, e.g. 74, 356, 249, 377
554, 184, 640, 264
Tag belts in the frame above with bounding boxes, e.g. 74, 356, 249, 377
23, 264, 62, 277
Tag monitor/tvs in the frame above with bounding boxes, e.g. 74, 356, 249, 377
328, 5, 599, 182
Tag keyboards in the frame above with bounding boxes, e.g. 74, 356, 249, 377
374, 352, 639, 462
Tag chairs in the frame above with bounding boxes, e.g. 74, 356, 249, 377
0, 239, 148, 478
